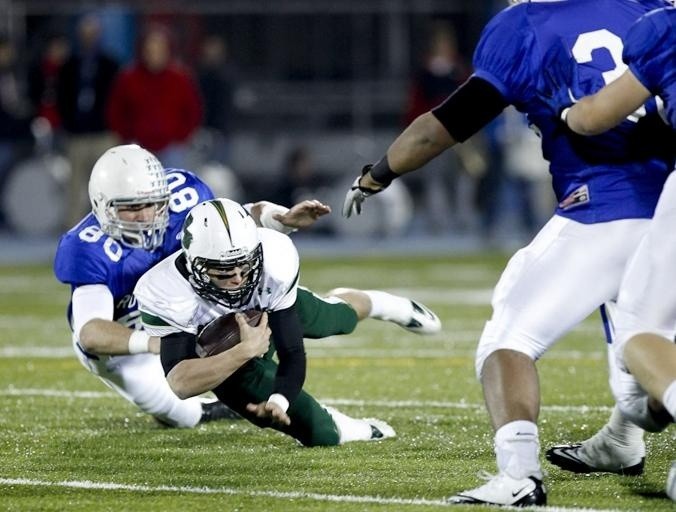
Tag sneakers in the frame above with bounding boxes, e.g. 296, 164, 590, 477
367, 290, 443, 336
322, 405, 396, 444
448, 467, 548, 507
199, 396, 243, 421
545, 425, 646, 476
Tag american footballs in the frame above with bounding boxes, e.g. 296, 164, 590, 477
195, 310, 263, 358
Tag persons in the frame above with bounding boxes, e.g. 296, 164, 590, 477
341, 0, 676, 508
407, 30, 555, 243
130, 198, 444, 449
542, 0, 676, 502
0, 0, 235, 230
52, 142, 332, 430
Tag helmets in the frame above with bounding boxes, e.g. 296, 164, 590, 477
181, 197, 264, 317
87, 142, 171, 254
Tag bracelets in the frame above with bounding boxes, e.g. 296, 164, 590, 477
128, 330, 150, 354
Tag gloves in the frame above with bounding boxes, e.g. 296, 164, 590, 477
532, 57, 600, 125
342, 163, 386, 219
